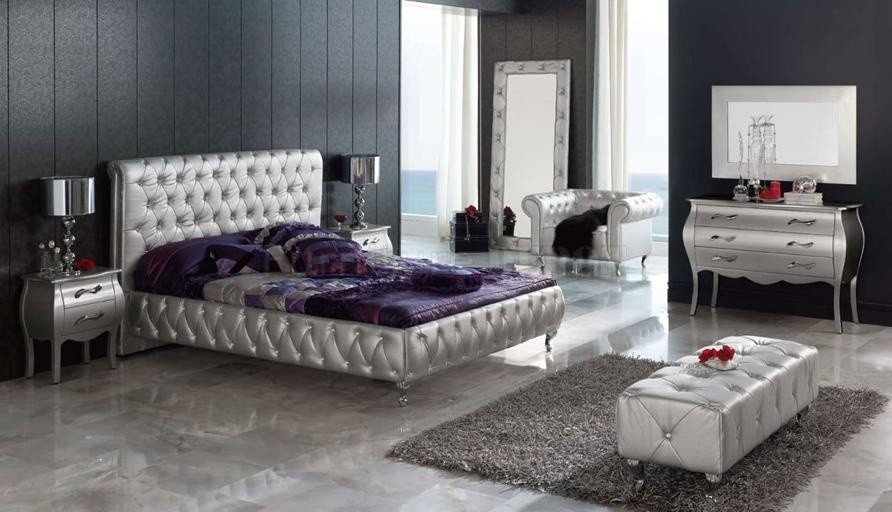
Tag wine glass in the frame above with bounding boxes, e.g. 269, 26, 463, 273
333, 209, 348, 231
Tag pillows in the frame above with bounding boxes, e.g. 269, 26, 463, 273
413, 261, 483, 294
208, 222, 378, 280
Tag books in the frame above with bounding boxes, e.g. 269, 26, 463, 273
784, 192, 824, 206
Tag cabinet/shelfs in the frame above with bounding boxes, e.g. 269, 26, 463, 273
680, 191, 862, 332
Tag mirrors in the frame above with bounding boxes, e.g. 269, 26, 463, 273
710, 85, 857, 185
485, 58, 572, 251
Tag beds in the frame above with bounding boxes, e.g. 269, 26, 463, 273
110, 149, 565, 409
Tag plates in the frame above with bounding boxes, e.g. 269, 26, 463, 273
756, 196, 784, 204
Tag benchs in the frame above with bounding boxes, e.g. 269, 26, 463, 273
615, 334, 824, 484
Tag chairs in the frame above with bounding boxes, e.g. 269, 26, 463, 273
523, 186, 663, 276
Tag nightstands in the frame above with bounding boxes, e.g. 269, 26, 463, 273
22, 265, 127, 385
330, 219, 393, 254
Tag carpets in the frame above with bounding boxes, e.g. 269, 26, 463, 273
381, 355, 892, 510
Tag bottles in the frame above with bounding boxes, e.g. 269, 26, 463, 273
38, 241, 64, 277
733, 176, 762, 202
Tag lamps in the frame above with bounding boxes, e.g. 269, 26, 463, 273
340, 152, 381, 230
43, 175, 96, 275
